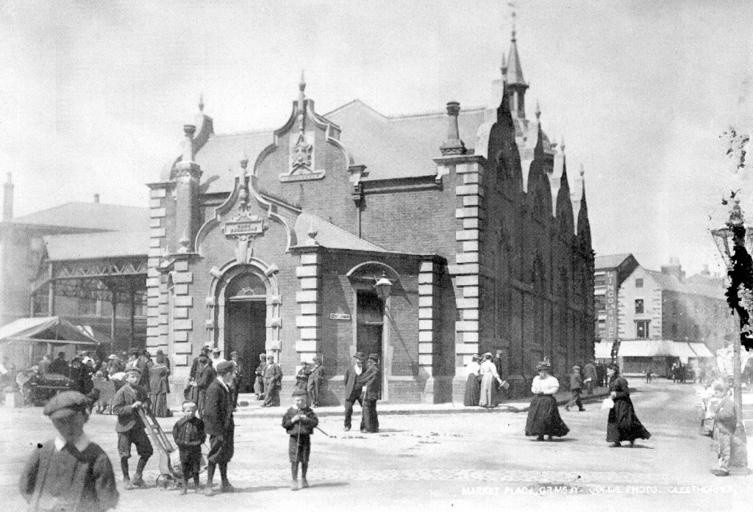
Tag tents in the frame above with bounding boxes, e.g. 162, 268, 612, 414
0, 317, 112, 347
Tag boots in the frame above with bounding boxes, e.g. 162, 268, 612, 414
219, 461, 234, 492
133, 454, 150, 488
204, 462, 216, 496
121, 457, 133, 490
193, 477, 205, 495
302, 461, 309, 489
178, 478, 188, 496
290, 461, 299, 491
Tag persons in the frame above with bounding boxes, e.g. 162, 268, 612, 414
209, 347, 226, 372
606, 363, 651, 447
156, 350, 171, 393
295, 361, 311, 391
19, 391, 120, 512
607, 363, 616, 388
188, 345, 212, 419
112, 348, 141, 371
305, 356, 325, 407
281, 389, 318, 490
1, 346, 112, 414
643, 366, 653, 384
203, 360, 239, 496
113, 366, 152, 490
260, 356, 282, 407
253, 353, 266, 400
111, 366, 128, 393
564, 365, 586, 411
583, 359, 597, 395
611, 361, 619, 381
172, 401, 206, 496
671, 363, 685, 383
464, 353, 483, 406
343, 351, 367, 431
694, 364, 735, 475
136, 351, 153, 399
478, 352, 510, 407
524, 361, 570, 441
357, 353, 381, 432
151, 355, 174, 417
228, 351, 243, 412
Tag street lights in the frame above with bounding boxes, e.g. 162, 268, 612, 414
711, 222, 753, 475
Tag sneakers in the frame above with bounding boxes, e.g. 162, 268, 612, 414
564, 404, 570, 412
345, 424, 350, 431
360, 425, 368, 434
578, 406, 586, 411
536, 434, 544, 441
609, 441, 622, 448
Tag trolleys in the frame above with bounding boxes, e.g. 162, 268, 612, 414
136, 403, 188, 488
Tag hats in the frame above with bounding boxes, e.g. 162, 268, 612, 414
537, 361, 550, 372
293, 390, 307, 396
607, 363, 620, 372
369, 352, 379, 360
125, 367, 141, 375
43, 390, 90, 415
215, 361, 234, 373
353, 351, 369, 360
